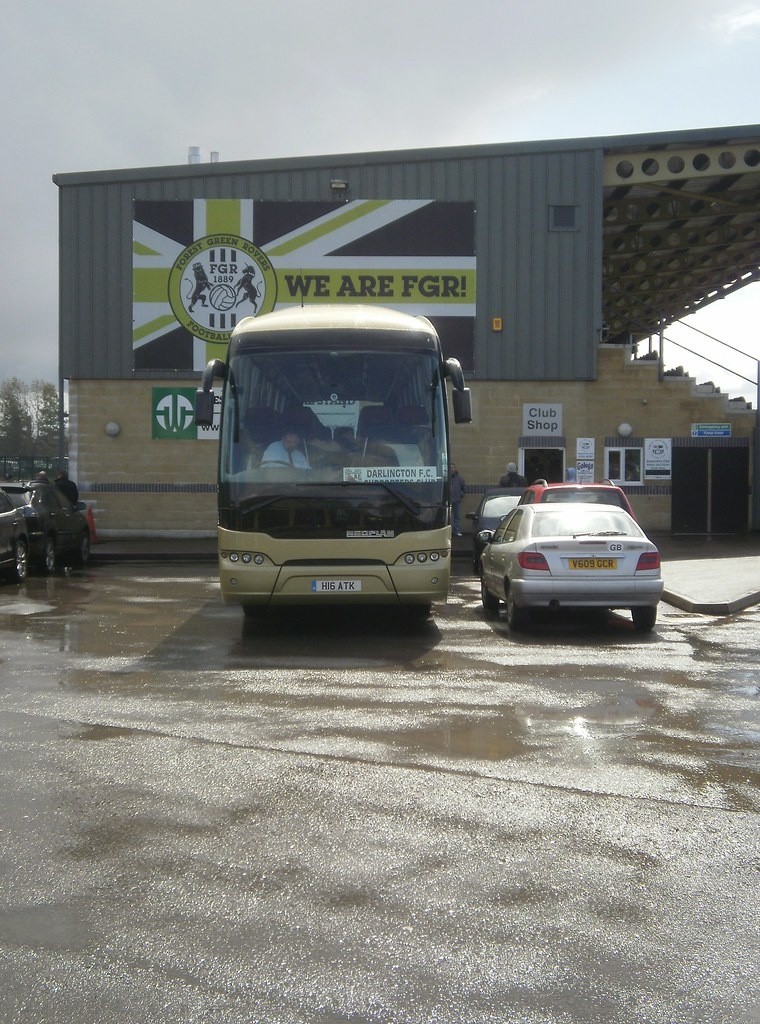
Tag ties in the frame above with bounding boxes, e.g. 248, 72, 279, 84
287, 451, 293, 467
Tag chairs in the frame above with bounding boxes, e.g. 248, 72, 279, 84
245, 406, 332, 469
334, 405, 437, 466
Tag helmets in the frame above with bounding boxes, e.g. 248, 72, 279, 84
506, 463, 517, 472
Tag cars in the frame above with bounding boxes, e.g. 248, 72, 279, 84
476, 501, 666, 632
0, 487, 30, 587
2, 478, 93, 571
465, 485, 534, 574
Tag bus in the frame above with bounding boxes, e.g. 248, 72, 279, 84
196, 303, 472, 627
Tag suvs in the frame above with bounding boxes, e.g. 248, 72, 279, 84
516, 476, 639, 526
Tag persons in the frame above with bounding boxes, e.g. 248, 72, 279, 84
450, 462, 466, 536
259, 429, 309, 468
499, 456, 545, 487
36, 468, 78, 504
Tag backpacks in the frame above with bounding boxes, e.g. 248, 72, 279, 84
507, 473, 521, 488
